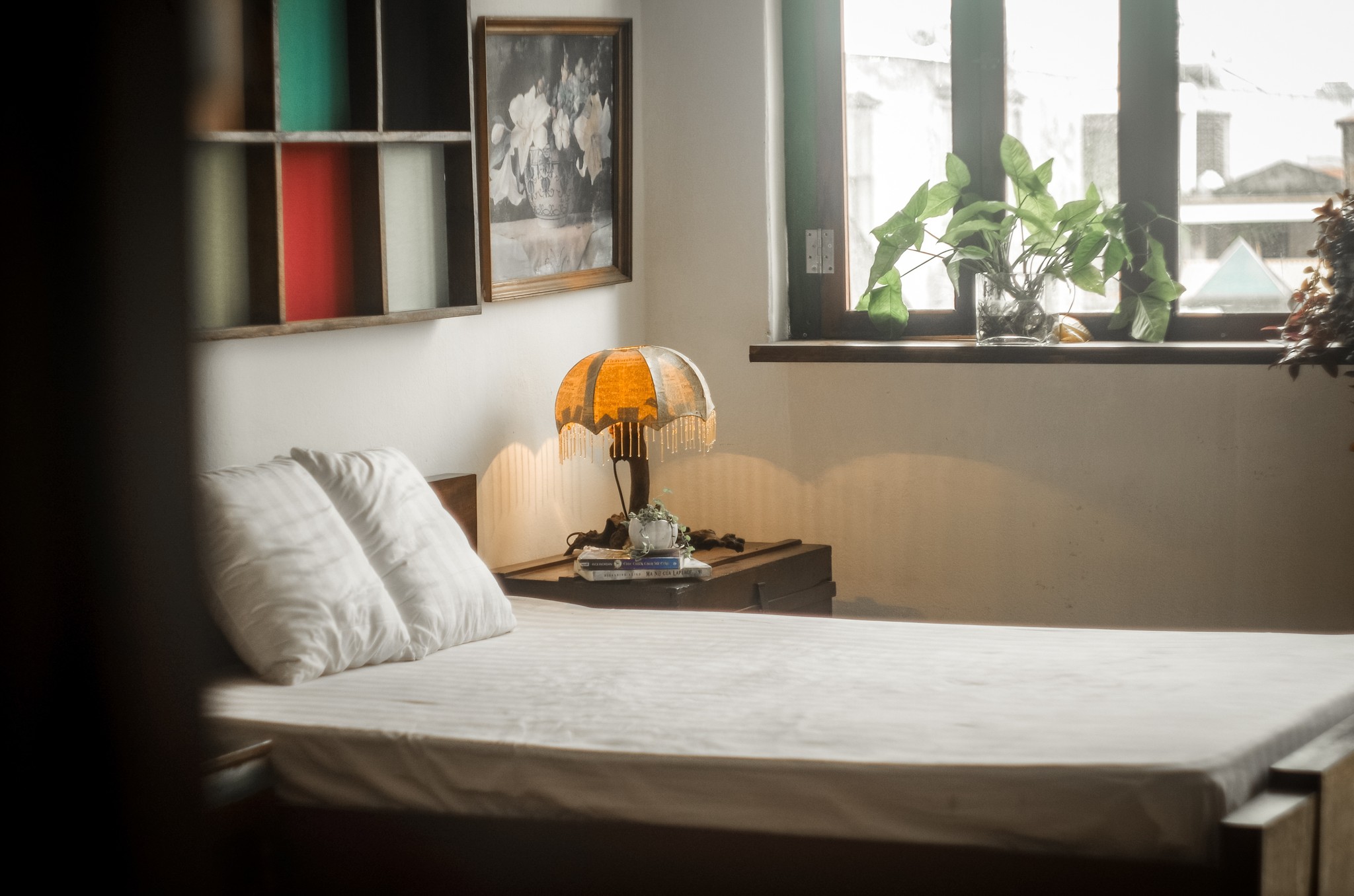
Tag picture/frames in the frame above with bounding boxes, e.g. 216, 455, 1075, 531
471, 16, 634, 303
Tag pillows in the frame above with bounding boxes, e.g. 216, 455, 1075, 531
291, 442, 518, 661
193, 454, 417, 685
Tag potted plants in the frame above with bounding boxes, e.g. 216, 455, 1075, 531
855, 133, 1187, 347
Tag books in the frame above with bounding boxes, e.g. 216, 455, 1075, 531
572, 557, 712, 581
580, 545, 692, 570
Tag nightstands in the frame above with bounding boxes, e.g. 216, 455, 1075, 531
493, 538, 837, 618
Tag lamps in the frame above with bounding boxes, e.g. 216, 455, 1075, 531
554, 346, 747, 557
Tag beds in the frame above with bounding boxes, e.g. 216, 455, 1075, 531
207, 593, 1353, 896
